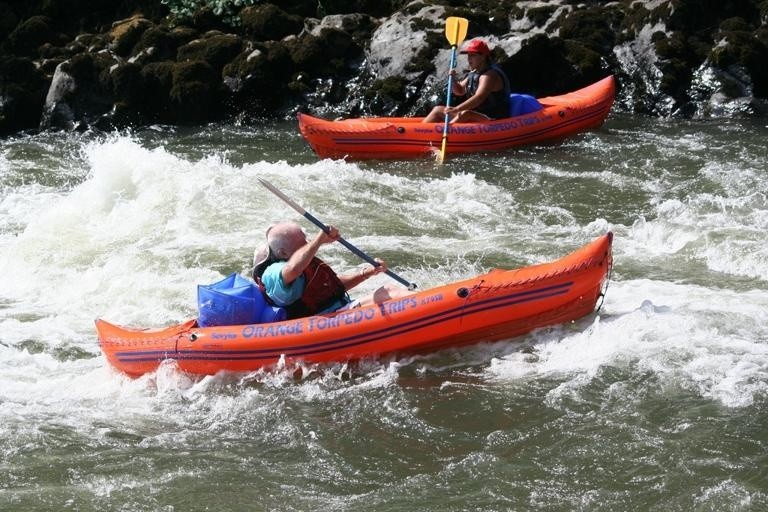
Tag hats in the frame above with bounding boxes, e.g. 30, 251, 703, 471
251, 226, 273, 285
458, 39, 489, 54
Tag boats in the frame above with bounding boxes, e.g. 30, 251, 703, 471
293, 74, 619, 164
92, 229, 621, 381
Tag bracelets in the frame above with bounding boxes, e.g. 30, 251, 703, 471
359, 266, 368, 281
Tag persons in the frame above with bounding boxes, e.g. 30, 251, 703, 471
260, 221, 413, 321
420, 38, 511, 125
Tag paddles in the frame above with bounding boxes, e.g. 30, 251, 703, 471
438, 17, 468, 164
255, 176, 418, 291
